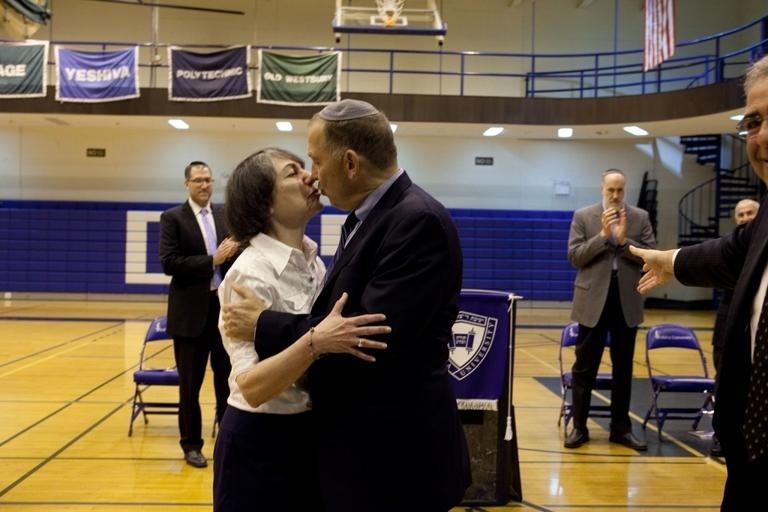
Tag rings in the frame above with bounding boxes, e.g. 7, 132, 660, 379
357, 338, 363, 350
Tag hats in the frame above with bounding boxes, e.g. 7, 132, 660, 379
316, 95, 382, 122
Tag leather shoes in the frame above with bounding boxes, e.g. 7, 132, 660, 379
564, 427, 590, 448
609, 430, 648, 451
185, 449, 208, 467
712, 438, 723, 457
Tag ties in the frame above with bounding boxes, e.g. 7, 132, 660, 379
310, 210, 361, 313
741, 286, 768, 462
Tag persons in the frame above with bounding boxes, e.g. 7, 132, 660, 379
562, 169, 658, 455
706, 199, 761, 458
211, 146, 393, 512
156, 160, 252, 470
625, 55, 766, 512
220, 98, 476, 512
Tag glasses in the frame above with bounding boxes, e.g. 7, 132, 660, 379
736, 112, 764, 140
187, 178, 215, 183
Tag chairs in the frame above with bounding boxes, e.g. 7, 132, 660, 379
558, 317, 636, 433
647, 311, 718, 442
121, 316, 201, 440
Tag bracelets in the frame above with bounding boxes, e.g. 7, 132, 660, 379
308, 327, 322, 361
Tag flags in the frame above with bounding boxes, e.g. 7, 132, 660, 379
53, 44, 142, 103
641, 0, 677, 71
0, 39, 50, 99
165, 42, 254, 103
251, 47, 342, 107
